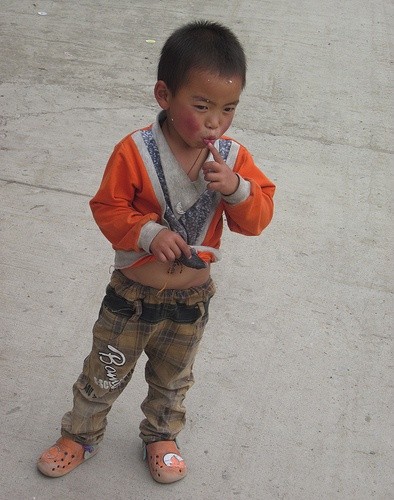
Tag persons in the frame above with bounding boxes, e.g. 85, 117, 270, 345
37, 21, 276, 483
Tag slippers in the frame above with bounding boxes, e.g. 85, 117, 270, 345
145, 439, 187, 484
36, 436, 101, 477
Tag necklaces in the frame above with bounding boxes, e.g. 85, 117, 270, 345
161, 116, 203, 174
